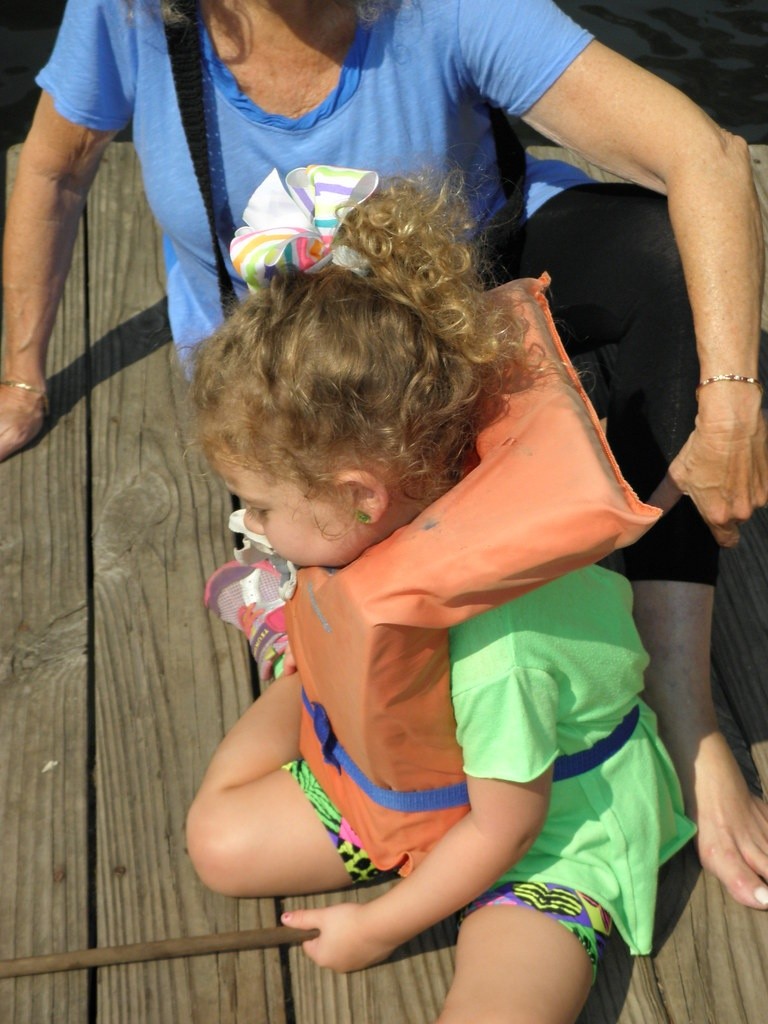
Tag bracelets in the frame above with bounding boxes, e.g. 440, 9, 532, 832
2, 377, 48, 408
693, 375, 763, 400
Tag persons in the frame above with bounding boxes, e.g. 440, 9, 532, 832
183, 166, 698, 1024
1, 0, 768, 908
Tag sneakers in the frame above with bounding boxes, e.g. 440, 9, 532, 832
202, 560, 299, 680
